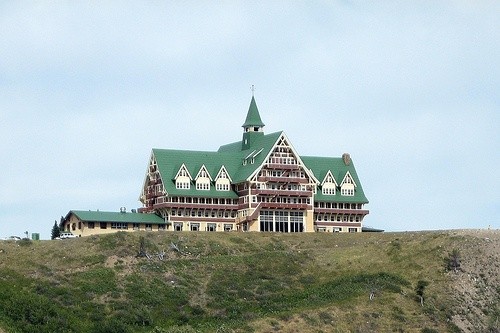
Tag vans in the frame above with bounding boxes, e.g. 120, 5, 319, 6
60, 233, 76, 239
